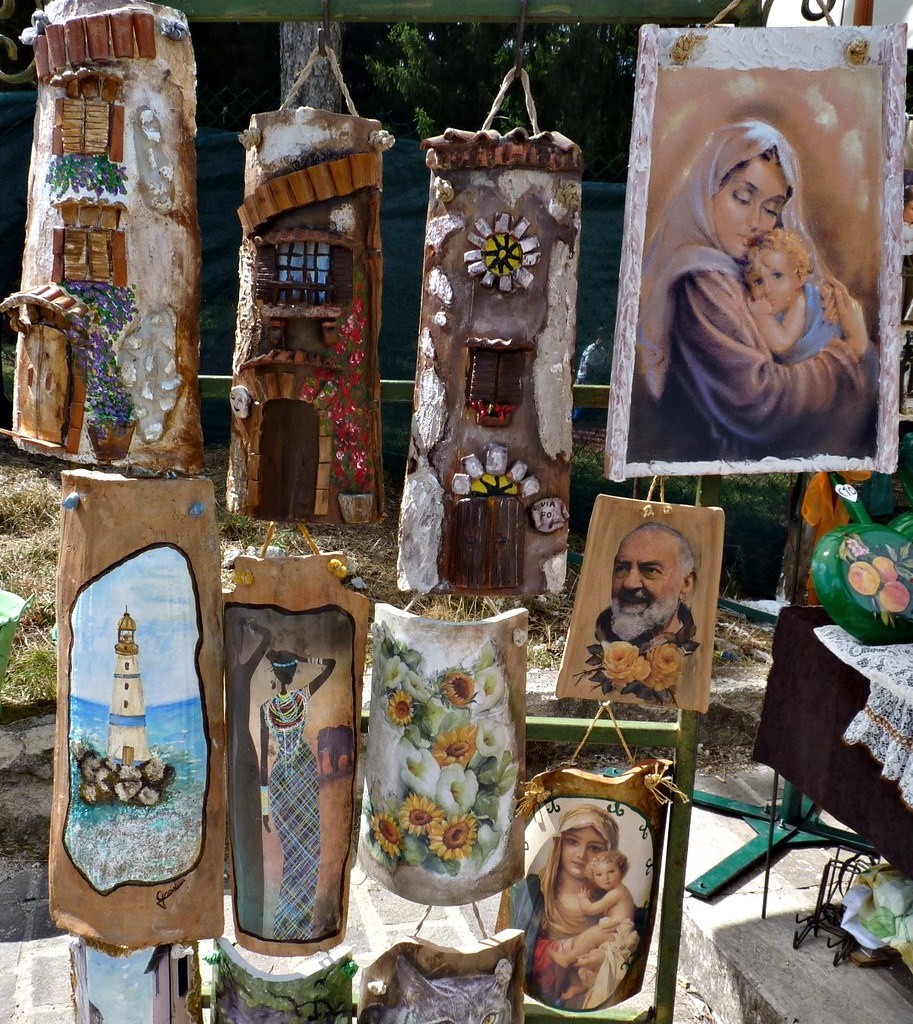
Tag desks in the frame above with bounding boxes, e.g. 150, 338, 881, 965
750, 606, 913, 920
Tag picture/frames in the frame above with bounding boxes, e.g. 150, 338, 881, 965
602, 22, 909, 484
